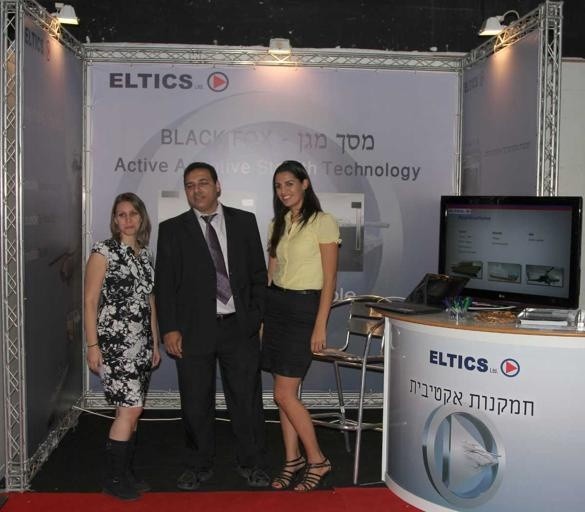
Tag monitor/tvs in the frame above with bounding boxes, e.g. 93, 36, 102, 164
439, 196, 583, 313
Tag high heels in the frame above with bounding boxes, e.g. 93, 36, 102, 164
270, 455, 309, 490
293, 459, 335, 492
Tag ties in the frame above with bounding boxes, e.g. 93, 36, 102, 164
200, 213, 233, 307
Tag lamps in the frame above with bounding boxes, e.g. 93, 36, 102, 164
49, 2, 77, 23
266, 34, 291, 55
478, 8, 520, 36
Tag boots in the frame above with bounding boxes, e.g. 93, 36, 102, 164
126, 430, 154, 493
101, 437, 141, 502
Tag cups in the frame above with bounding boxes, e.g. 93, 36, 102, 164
444, 295, 471, 320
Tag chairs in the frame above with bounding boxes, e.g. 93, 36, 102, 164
298, 293, 392, 485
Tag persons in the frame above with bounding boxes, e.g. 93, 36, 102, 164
153, 163, 272, 491
82, 193, 162, 500
259, 161, 343, 492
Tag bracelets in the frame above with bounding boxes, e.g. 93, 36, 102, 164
86, 342, 99, 348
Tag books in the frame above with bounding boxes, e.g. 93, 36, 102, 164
518, 309, 572, 327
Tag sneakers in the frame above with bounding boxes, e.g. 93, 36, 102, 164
175, 466, 214, 491
234, 464, 272, 488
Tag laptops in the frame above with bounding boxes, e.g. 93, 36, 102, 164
365, 272, 469, 315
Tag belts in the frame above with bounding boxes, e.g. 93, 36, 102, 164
265, 280, 322, 296
215, 312, 237, 321
